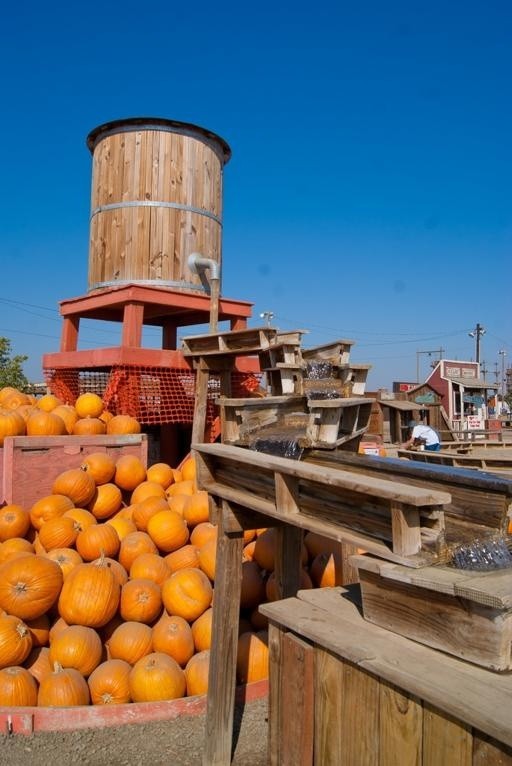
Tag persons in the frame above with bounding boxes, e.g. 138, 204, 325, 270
403, 420, 440, 451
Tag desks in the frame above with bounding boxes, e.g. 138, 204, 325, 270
258, 586, 511, 766
42, 283, 261, 418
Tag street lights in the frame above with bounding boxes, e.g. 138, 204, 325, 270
260, 311, 276, 327
469, 323, 487, 362
497, 349, 508, 408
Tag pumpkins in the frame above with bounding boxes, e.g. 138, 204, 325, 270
0, 385, 142, 446
1, 453, 365, 706
